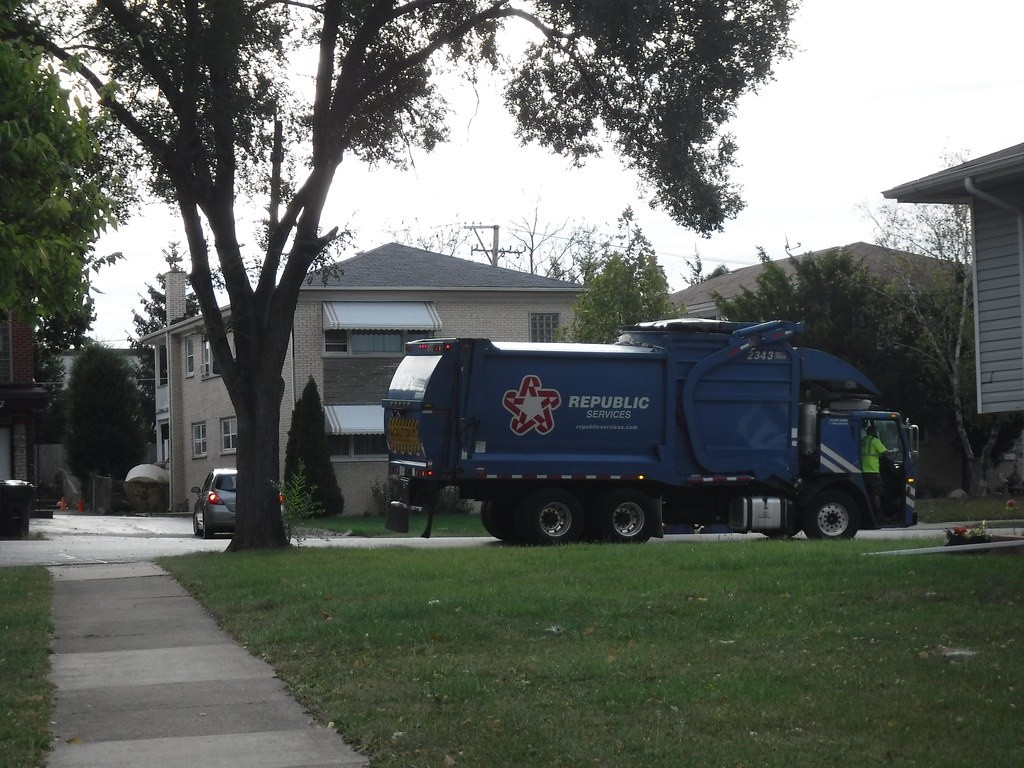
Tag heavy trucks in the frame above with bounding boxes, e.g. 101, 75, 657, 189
383, 317, 920, 545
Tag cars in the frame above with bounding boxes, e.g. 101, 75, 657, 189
191, 467, 284, 540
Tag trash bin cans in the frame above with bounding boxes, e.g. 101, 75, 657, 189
0, 480, 35, 537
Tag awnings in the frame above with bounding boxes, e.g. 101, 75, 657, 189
323, 405, 384, 435
323, 301, 442, 334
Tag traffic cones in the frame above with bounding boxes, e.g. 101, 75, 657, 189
60, 497, 66, 511
78, 498, 84, 512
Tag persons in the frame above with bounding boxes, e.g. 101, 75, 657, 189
860, 426, 900, 523
